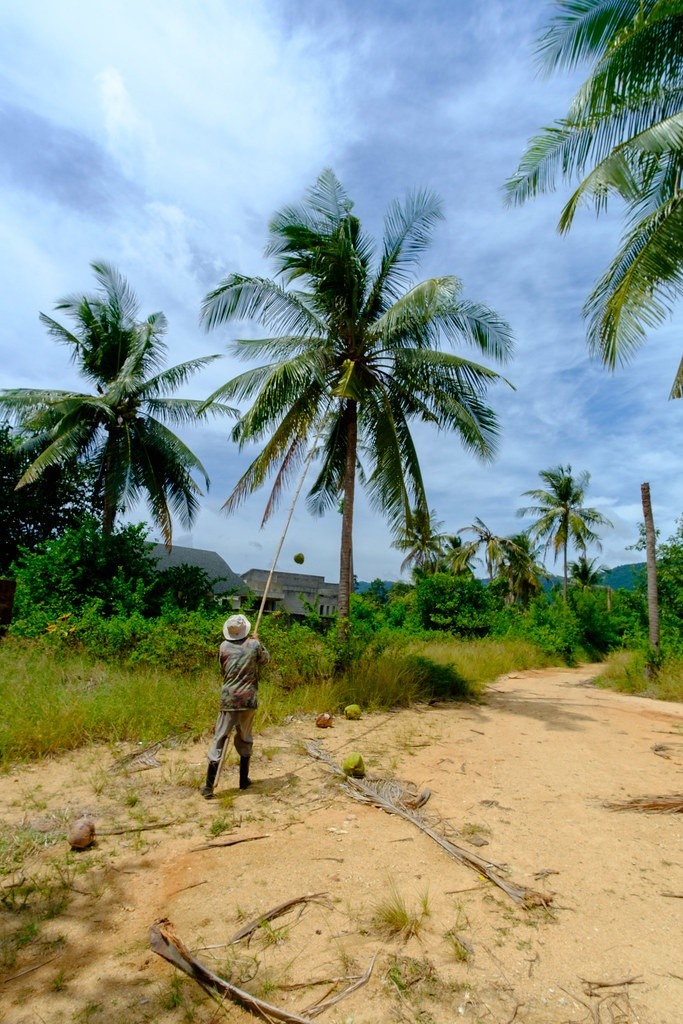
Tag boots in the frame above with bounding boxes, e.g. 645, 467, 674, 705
200, 761, 219, 798
239, 756, 252, 790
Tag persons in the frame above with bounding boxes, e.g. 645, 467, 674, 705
201, 614, 269, 799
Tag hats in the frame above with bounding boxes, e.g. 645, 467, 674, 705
223, 614, 252, 640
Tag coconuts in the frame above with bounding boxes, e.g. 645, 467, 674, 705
294, 553, 305, 563
66, 815, 99, 849
313, 704, 364, 774
